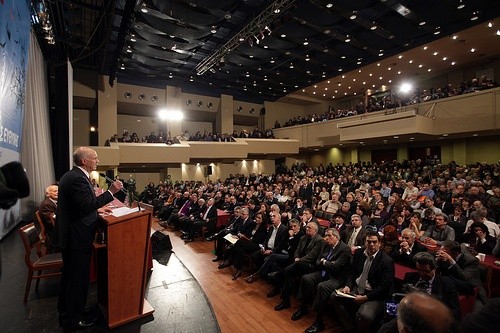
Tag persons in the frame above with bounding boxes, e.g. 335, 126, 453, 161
330, 231, 395, 333
283, 74, 494, 128
244, 219, 305, 298
56, 147, 123, 330
205, 197, 319, 281
276, 160, 500, 191
181, 130, 234, 142
290, 228, 352, 333
110, 131, 179, 145
141, 172, 329, 243
273, 120, 281, 128
113, 174, 136, 202
322, 179, 500, 294
398, 291, 451, 333
233, 127, 276, 138
267, 222, 324, 312
39, 185, 62, 248
378, 253, 457, 333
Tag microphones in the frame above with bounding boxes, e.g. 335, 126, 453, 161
99, 172, 140, 211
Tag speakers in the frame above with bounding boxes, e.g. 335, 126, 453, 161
151, 230, 173, 255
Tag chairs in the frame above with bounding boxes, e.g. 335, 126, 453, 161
17, 222, 64, 305
36, 208, 47, 255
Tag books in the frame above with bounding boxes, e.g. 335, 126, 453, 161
334, 289, 356, 299
99, 206, 145, 218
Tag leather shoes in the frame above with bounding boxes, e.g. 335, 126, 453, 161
274, 301, 290, 311
291, 307, 307, 320
65, 314, 98, 331
305, 321, 324, 333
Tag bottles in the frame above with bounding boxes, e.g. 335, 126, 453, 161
373, 225, 377, 231
95, 230, 105, 244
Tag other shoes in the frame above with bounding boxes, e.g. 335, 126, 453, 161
233, 271, 241, 280
267, 289, 280, 297
219, 261, 232, 269
244, 273, 260, 283
177, 227, 194, 242
206, 236, 215, 241
212, 256, 223, 262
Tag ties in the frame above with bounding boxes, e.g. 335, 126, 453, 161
357, 256, 372, 294
321, 249, 333, 277
348, 230, 356, 246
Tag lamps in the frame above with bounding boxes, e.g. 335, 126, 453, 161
265, 25, 274, 35
247, 37, 254, 46
252, 35, 260, 44
220, 56, 227, 63
33, 8, 58, 45
258, 30, 265, 40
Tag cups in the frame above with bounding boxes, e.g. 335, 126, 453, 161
479, 253, 486, 261
